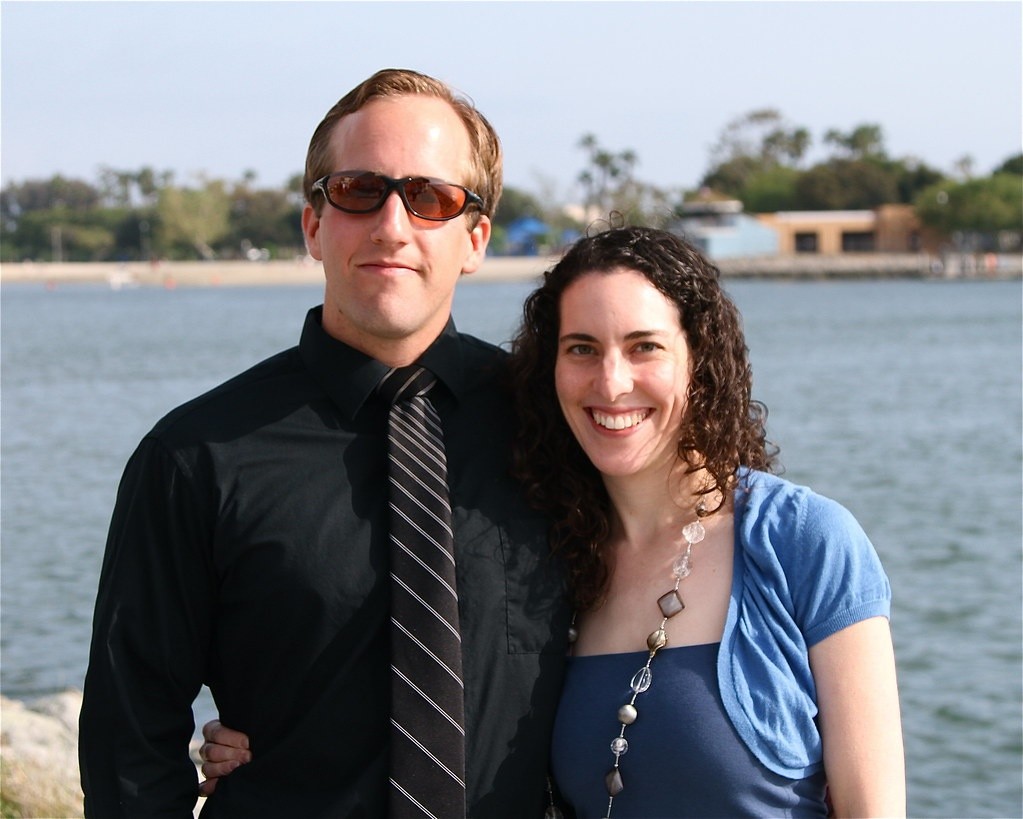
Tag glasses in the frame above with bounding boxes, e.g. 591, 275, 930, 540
312, 170, 484, 221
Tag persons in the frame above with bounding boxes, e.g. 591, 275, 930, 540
76, 67, 612, 819
193, 212, 908, 819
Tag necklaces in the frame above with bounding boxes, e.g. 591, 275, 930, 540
547, 461, 722, 819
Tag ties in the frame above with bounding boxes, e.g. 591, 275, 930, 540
376, 365, 467, 819
